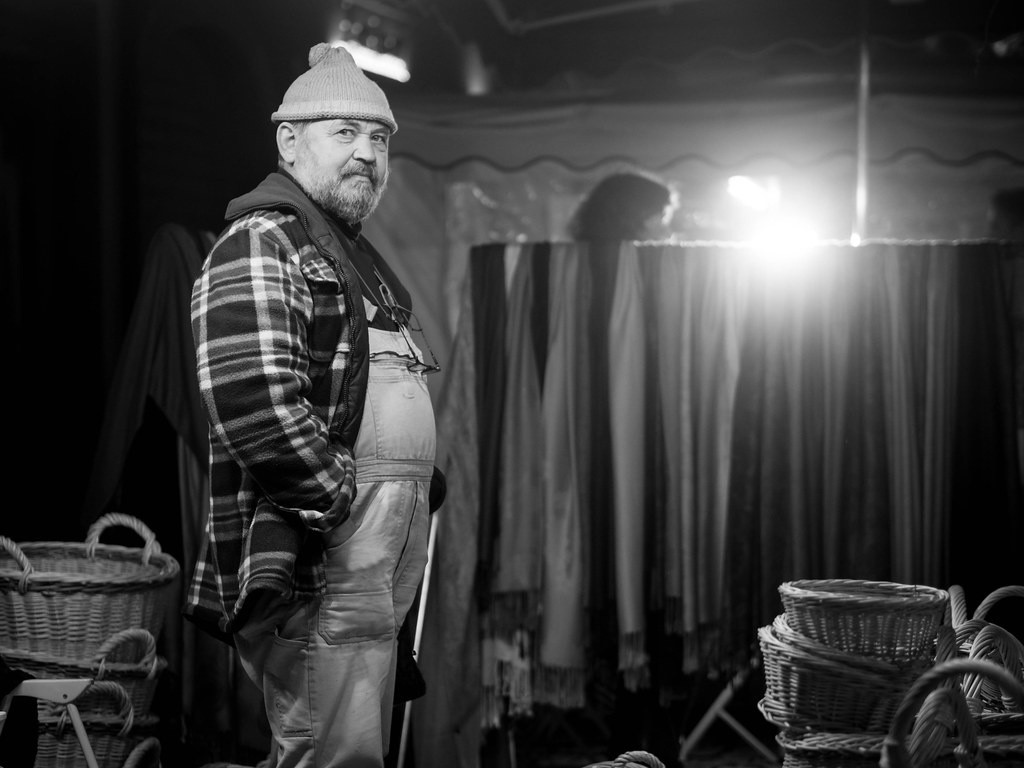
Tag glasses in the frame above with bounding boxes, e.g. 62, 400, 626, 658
375, 302, 442, 375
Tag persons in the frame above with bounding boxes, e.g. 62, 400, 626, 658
566, 170, 671, 244
190, 44, 438, 768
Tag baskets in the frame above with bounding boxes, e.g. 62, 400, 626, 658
0, 512, 180, 768
756, 579, 1024, 768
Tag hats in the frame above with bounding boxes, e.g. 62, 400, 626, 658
272, 43, 398, 137
